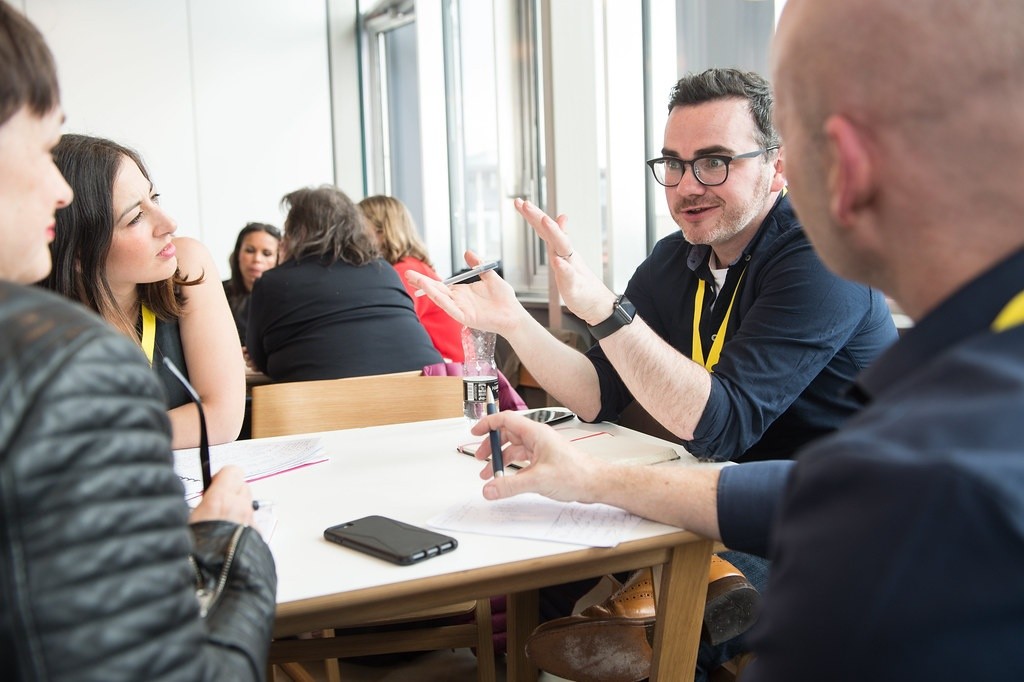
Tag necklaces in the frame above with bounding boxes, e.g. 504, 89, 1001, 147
140, 303, 156, 368
690, 267, 745, 371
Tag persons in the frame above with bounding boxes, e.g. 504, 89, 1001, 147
221, 188, 473, 382
410, 71, 896, 682
41, 135, 246, 449
0, 1, 277, 682
468, 0, 1024, 682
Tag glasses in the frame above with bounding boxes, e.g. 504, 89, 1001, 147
248, 223, 278, 234
646, 146, 779, 187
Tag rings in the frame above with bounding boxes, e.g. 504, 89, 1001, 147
557, 247, 576, 260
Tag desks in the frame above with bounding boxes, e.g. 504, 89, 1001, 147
172, 408, 738, 682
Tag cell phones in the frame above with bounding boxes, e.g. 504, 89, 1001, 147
323, 516, 458, 565
523, 409, 575, 425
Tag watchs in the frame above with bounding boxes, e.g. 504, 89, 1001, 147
586, 293, 637, 341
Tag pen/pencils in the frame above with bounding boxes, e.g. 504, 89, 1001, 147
486, 384, 505, 478
415, 261, 499, 297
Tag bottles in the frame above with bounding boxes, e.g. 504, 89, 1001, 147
461, 326, 499, 424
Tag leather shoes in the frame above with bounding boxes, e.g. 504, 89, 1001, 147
524, 555, 762, 682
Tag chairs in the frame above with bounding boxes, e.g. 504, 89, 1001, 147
252, 377, 496, 682
